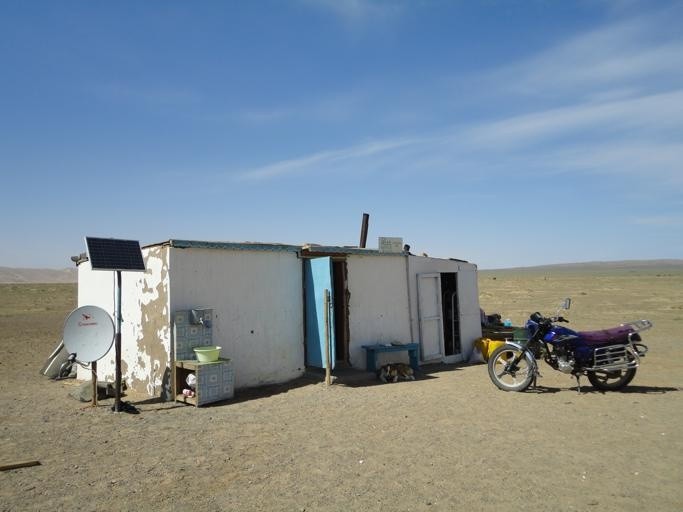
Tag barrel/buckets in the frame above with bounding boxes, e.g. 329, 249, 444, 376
476, 338, 490, 364
489, 340, 507, 365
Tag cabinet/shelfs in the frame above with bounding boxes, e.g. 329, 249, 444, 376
175, 359, 235, 408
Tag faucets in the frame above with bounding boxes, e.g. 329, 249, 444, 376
198, 317, 205, 327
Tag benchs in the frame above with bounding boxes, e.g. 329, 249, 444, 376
361, 344, 419, 373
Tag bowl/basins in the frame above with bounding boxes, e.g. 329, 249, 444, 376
192, 346, 223, 364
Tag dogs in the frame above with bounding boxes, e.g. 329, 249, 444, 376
379, 363, 416, 384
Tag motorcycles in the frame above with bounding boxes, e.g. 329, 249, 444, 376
487, 298, 651, 393
